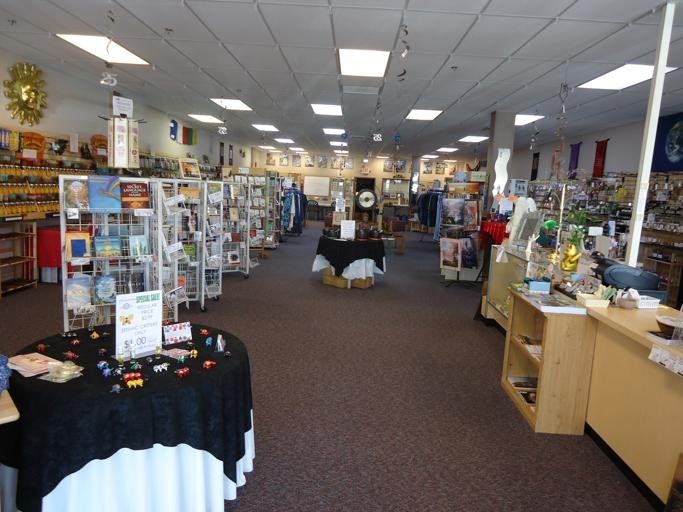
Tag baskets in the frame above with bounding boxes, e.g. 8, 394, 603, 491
577, 294, 610, 307
638, 296, 660, 308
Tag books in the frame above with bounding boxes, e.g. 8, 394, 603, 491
507, 335, 542, 406
63, 272, 116, 310
8, 352, 63, 378
64, 174, 149, 208
46, 213, 60, 218
65, 230, 148, 258
0, 128, 22, 152
0, 216, 23, 222
522, 281, 586, 314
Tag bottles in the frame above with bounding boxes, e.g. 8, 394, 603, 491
1, 166, 96, 215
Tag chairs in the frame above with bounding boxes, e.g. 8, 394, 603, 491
306, 200, 320, 220
329, 201, 336, 211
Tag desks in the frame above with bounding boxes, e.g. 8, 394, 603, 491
312, 234, 386, 290
0, 321, 255, 512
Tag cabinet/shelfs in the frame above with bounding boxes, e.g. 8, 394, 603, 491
501, 285, 597, 436
526, 169, 683, 309
0, 125, 303, 331
